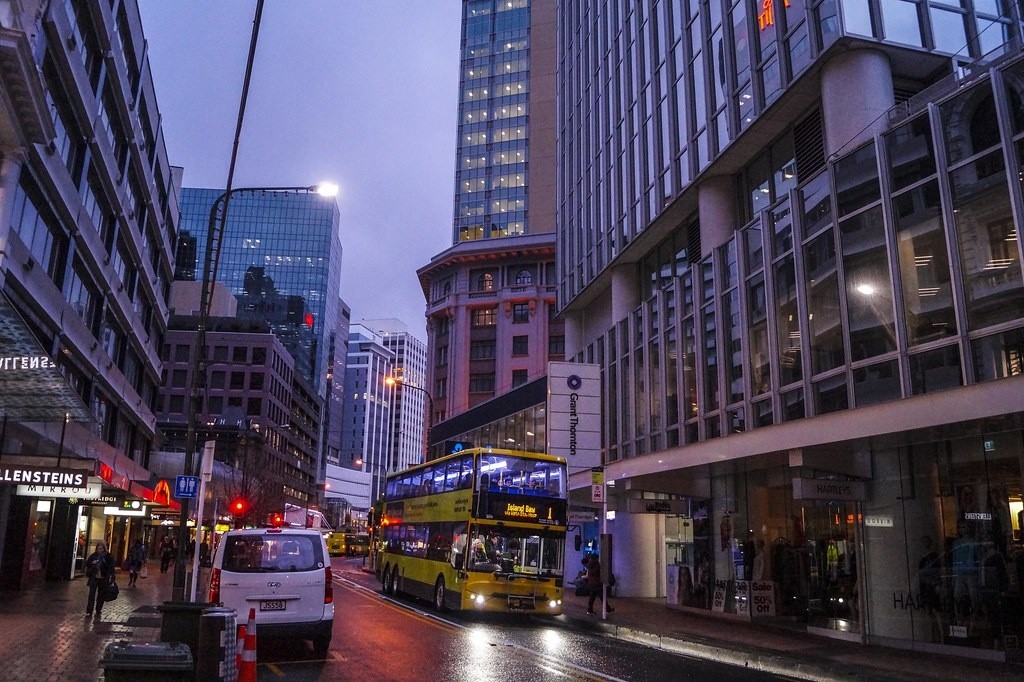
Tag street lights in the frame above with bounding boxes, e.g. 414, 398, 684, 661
162, 180, 342, 651
384, 376, 433, 462
355, 459, 387, 482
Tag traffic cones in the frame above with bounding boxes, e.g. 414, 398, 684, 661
236, 607, 258, 682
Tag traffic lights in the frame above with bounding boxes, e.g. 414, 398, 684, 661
234, 499, 244, 518
273, 512, 282, 525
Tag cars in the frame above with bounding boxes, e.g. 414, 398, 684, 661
914, 539, 1024, 624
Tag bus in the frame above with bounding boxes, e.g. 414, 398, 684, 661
374, 446, 583, 621
326, 532, 370, 558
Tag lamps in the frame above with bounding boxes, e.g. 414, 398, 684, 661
23, 32, 175, 423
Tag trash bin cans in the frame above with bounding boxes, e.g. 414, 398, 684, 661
98, 641, 195, 682
154, 600, 223, 671
195, 607, 238, 682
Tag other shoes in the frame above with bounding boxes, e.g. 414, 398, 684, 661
85, 613, 91, 618
133, 585, 136, 587
607, 608, 615, 615
164, 571, 166, 573
97, 611, 101, 614
587, 612, 596, 616
128, 582, 132, 585
161, 570, 163, 572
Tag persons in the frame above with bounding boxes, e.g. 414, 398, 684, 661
472, 538, 485, 561
497, 479, 503, 487
753, 540, 769, 581
581, 559, 588, 579
679, 566, 693, 606
843, 538, 861, 634
949, 518, 985, 635
200, 539, 208, 567
189, 540, 195, 561
486, 536, 500, 560
505, 479, 512, 487
160, 537, 172, 574
127, 539, 147, 587
462, 474, 471, 489
586, 553, 614, 616
85, 541, 115, 616
917, 536, 946, 644
529, 478, 536, 488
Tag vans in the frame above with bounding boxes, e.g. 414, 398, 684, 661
207, 526, 336, 654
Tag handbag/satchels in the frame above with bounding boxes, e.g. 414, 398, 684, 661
104, 581, 119, 602
141, 566, 148, 577
86, 565, 95, 578
